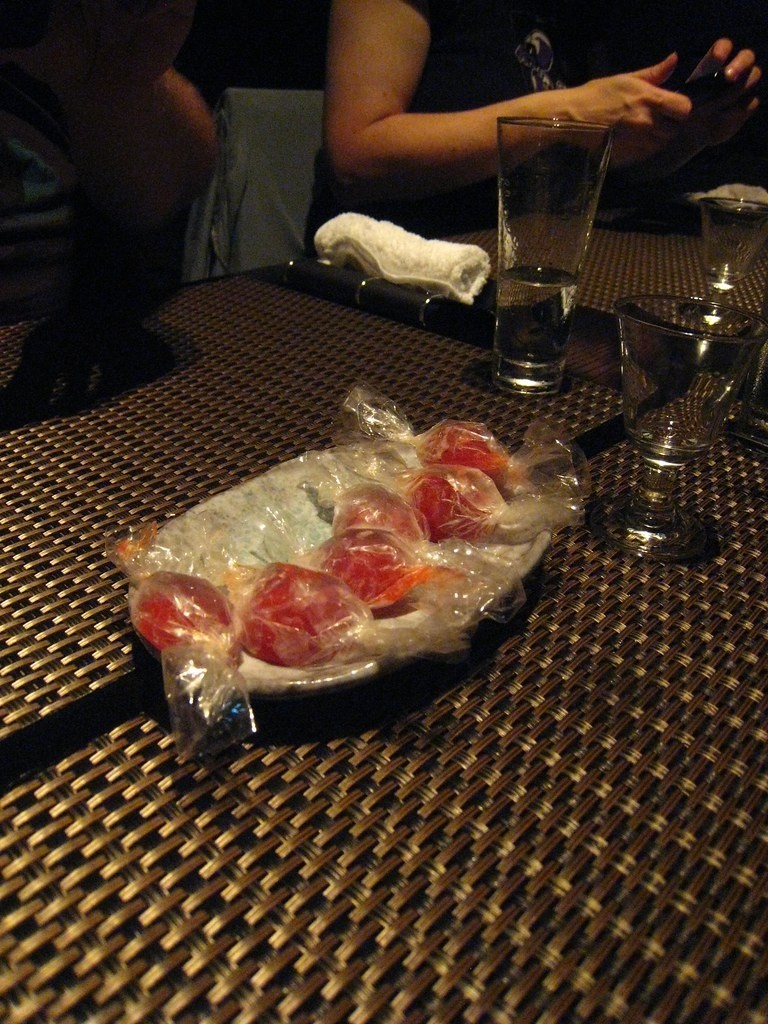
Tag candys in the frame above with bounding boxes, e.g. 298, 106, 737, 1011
104, 381, 591, 761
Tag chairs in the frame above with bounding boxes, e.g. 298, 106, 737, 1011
180, 88, 324, 282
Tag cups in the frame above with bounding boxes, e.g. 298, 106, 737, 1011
489, 115, 612, 396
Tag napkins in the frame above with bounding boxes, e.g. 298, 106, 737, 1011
687, 184, 768, 206
315, 211, 491, 305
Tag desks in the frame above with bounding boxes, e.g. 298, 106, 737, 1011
1, 192, 768, 1023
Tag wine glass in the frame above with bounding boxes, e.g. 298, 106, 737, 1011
588, 294, 768, 565
700, 193, 768, 305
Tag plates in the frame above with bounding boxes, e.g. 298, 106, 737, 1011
128, 442, 552, 702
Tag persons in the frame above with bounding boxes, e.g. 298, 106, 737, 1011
304, 0, 762, 258
